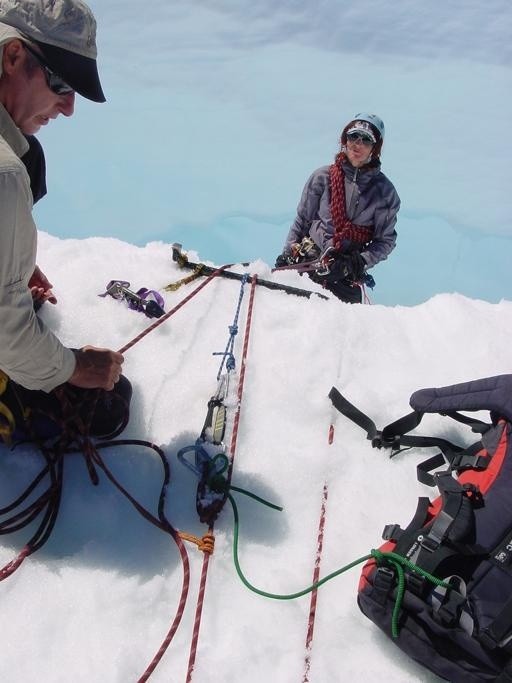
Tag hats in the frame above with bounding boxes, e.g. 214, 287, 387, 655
347, 121, 378, 144
0, 0, 106, 102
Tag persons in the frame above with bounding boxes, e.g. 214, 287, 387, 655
275, 113, 401, 304
0, 0, 132, 441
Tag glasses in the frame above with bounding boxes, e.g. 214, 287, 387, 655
346, 134, 372, 146
25, 48, 74, 96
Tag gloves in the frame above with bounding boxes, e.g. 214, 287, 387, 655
321, 251, 365, 283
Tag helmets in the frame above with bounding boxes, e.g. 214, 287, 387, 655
340, 113, 386, 151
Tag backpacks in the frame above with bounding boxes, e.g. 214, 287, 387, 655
358, 374, 512, 681
